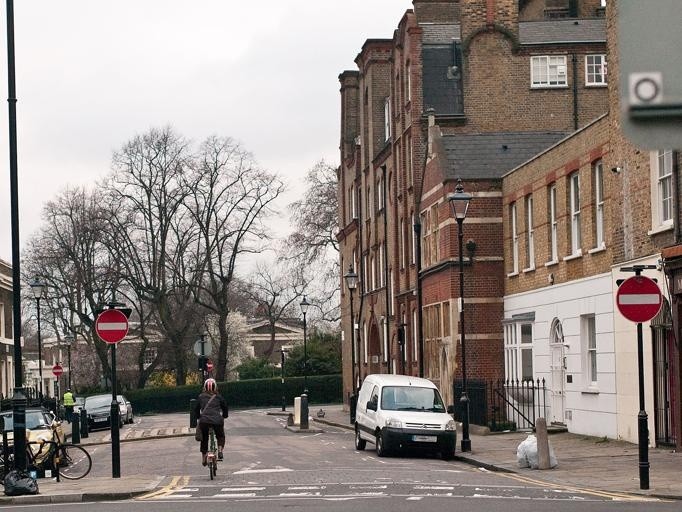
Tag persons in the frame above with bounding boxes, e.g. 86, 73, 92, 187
195, 378, 229, 466
62, 388, 77, 423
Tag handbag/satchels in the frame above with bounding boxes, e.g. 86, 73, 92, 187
194, 421, 202, 441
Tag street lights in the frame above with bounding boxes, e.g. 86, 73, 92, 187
300, 294, 309, 398
65, 331, 75, 390
344, 262, 360, 424
30, 273, 46, 408
448, 177, 474, 450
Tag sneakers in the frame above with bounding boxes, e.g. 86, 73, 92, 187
202, 453, 224, 466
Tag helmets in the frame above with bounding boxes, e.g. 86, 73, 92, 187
203, 378, 217, 392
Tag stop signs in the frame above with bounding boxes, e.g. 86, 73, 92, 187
614, 264, 665, 489
53, 359, 63, 400
95, 301, 133, 478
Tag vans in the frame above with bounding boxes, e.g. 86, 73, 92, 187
354, 373, 457, 462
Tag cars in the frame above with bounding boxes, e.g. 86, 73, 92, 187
84, 393, 135, 433
1, 409, 68, 481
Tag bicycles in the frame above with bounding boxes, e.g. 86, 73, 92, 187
0, 410, 93, 487
206, 427, 218, 479
